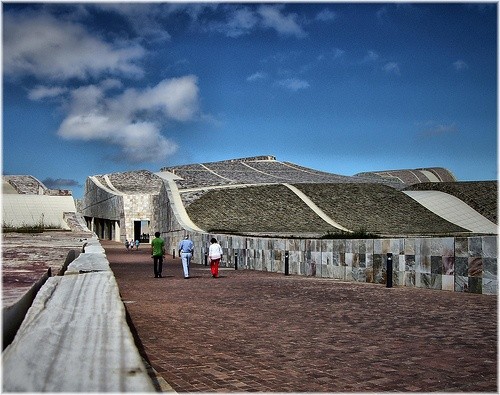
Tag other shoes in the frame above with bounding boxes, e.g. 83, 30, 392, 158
184, 276, 190, 279
154, 273, 162, 278
211, 274, 220, 278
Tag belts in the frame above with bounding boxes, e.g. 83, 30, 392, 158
182, 252, 190, 253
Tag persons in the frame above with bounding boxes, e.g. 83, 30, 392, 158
179, 236, 194, 279
150, 232, 165, 278
136, 240, 139, 251
130, 239, 134, 249
125, 240, 129, 249
209, 238, 223, 278
